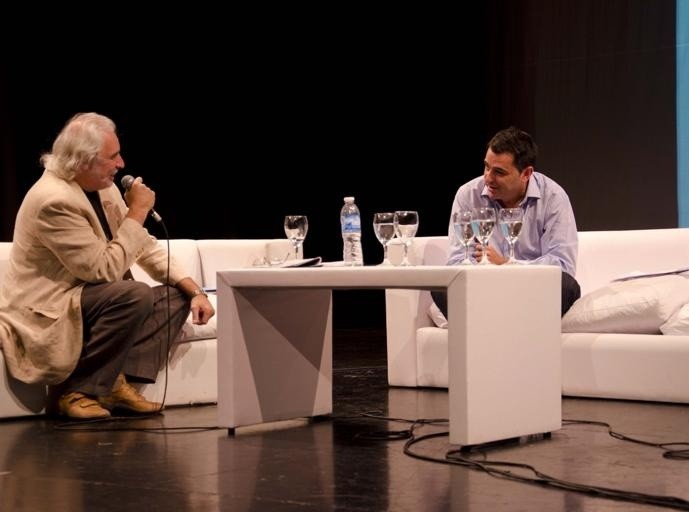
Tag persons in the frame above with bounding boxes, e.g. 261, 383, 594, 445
430, 126, 579, 321
1, 110, 216, 420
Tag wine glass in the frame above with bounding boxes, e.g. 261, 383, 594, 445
283, 214, 309, 259
373, 212, 394, 267
472, 205, 496, 263
453, 211, 475, 265
394, 211, 420, 266
499, 205, 523, 265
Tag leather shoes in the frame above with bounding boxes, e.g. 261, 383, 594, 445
44, 390, 112, 422
97, 371, 167, 415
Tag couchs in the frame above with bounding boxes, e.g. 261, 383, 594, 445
1, 238, 305, 422
381, 227, 689, 407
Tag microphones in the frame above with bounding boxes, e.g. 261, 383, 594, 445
121, 174, 162, 222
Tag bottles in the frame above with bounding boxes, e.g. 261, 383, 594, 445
340, 196, 365, 266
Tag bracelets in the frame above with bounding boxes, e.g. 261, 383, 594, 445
189, 286, 208, 299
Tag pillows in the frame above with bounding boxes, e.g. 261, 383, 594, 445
180, 291, 217, 341
658, 303, 689, 333
562, 274, 688, 333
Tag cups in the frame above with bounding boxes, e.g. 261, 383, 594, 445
263, 241, 289, 264
